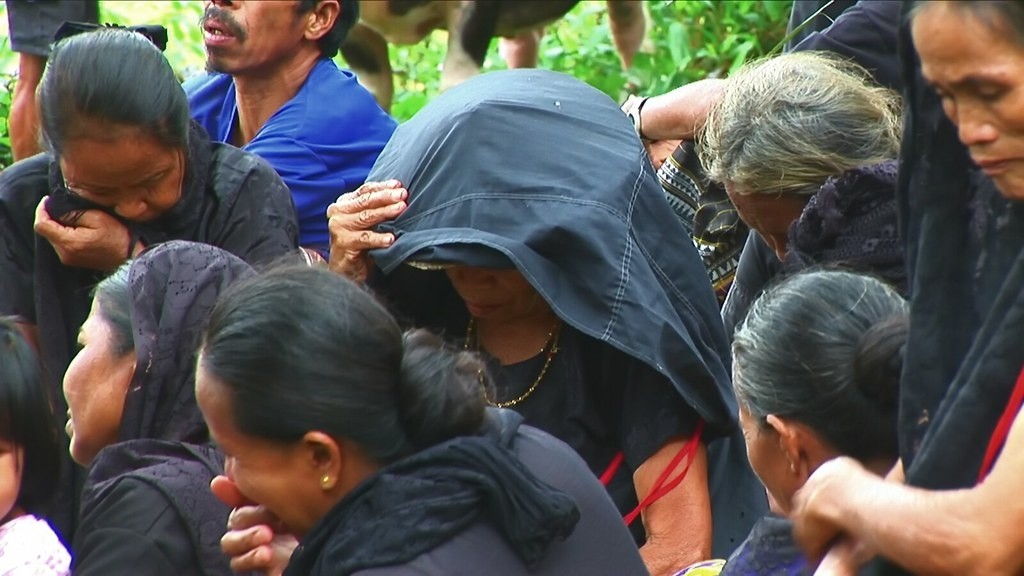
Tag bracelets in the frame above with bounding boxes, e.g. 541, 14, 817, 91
620, 94, 649, 139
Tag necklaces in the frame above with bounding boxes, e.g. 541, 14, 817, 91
463, 316, 561, 407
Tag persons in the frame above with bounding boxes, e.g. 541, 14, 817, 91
0, 1, 1024, 576
